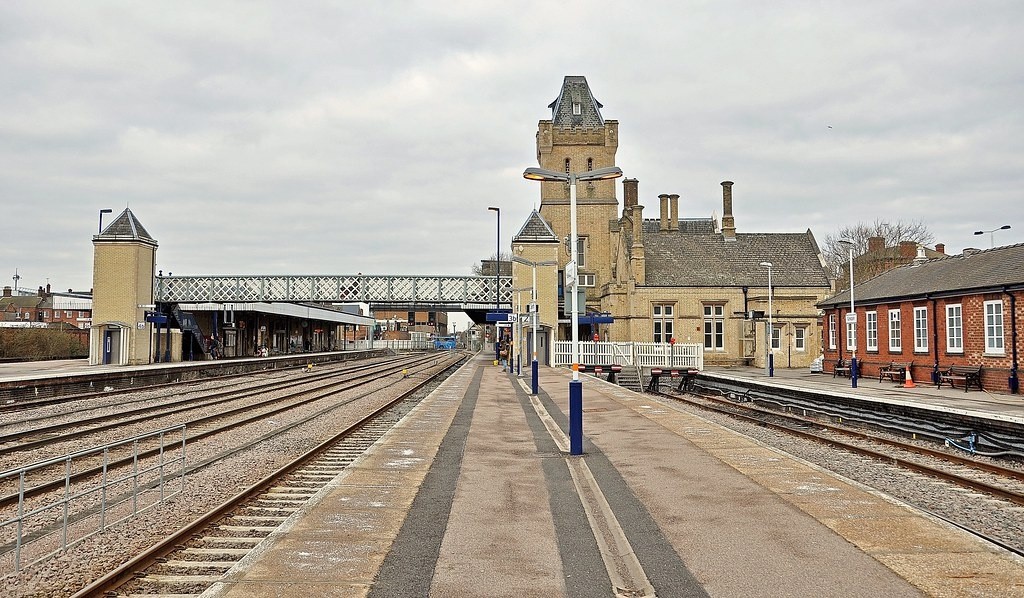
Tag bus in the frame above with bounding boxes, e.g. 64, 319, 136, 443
434, 340, 456, 350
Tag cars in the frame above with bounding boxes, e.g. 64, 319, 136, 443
810, 354, 824, 373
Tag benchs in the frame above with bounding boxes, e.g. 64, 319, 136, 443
878, 360, 915, 385
832, 357, 862, 379
936, 363, 983, 393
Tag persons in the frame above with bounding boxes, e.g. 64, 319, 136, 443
209, 336, 223, 360
305, 339, 309, 350
498, 340, 507, 372
290, 340, 295, 355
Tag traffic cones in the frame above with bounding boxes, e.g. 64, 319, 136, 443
904, 365, 916, 389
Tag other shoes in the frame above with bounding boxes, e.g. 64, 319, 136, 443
501, 369, 506, 372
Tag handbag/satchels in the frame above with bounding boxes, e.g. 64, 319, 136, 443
500, 350, 507, 357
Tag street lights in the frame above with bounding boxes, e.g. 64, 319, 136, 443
523, 165, 624, 455
512, 256, 561, 395
61, 319, 65, 332
502, 287, 534, 376
838, 238, 857, 389
973, 225, 1011, 248
99, 208, 113, 233
46, 278, 49, 284
760, 261, 775, 377
452, 322, 456, 350
488, 207, 500, 365
